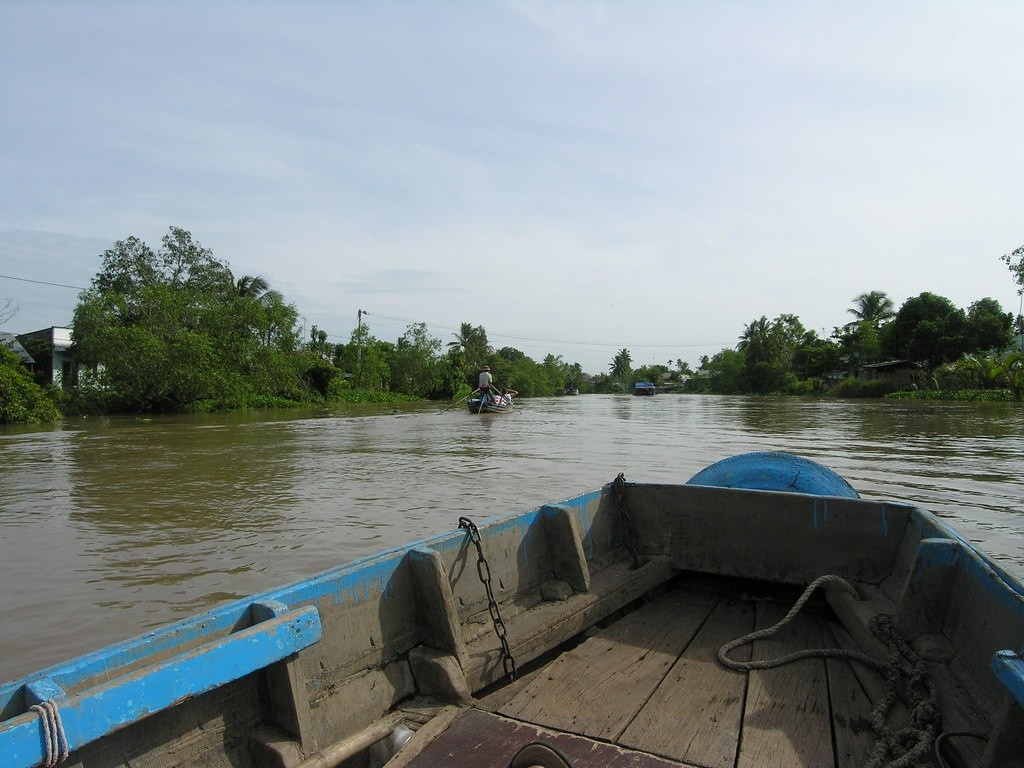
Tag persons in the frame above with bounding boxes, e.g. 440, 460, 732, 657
479, 364, 496, 402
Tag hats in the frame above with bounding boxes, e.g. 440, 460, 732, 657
482, 365, 491, 372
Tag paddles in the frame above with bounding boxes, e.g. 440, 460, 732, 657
440, 387, 480, 414
490, 384, 522, 415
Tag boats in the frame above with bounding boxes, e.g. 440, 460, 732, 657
568, 389, 579, 395
633, 382, 656, 395
0, 453, 1024, 767
554, 389, 567, 395
468, 399, 513, 414
